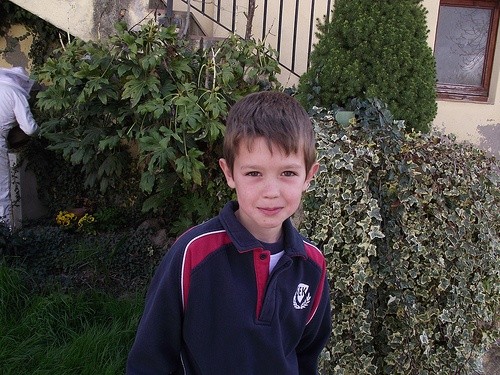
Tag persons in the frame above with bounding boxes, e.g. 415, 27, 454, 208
0, 66, 41, 229
126, 91, 330, 375
